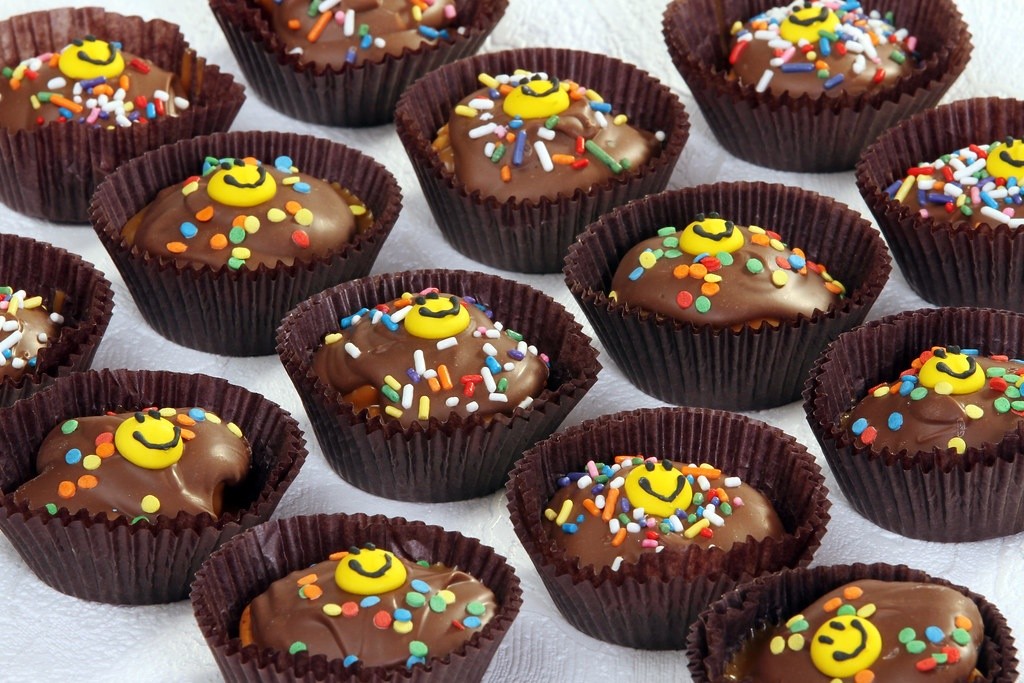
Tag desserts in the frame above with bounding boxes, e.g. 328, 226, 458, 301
0, 0, 1024, 683
